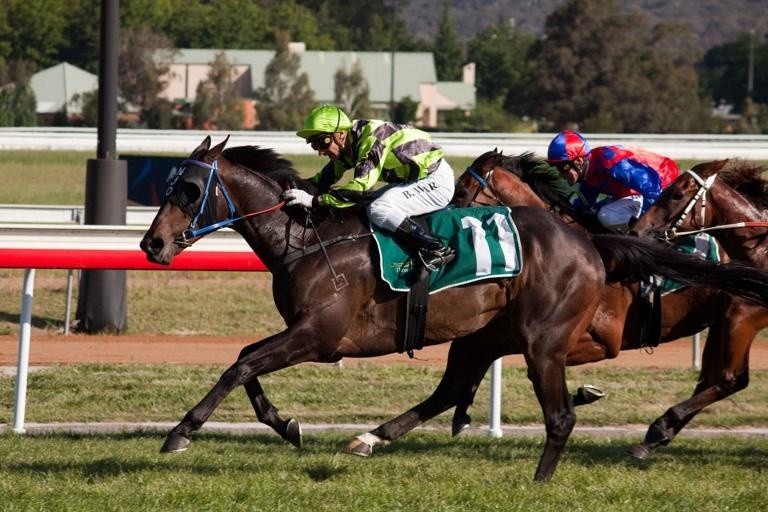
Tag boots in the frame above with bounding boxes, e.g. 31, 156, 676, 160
395, 217, 455, 284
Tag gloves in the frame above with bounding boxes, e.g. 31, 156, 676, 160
283, 190, 321, 210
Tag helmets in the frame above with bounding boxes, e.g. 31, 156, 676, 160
544, 130, 590, 166
296, 105, 353, 137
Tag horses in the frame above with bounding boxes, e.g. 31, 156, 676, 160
137, 133, 768, 484
625, 157, 768, 463
449, 144, 733, 439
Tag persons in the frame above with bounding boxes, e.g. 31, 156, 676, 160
277, 100, 458, 285
544, 124, 685, 300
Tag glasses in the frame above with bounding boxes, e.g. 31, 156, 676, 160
310, 134, 333, 150
557, 161, 576, 174
